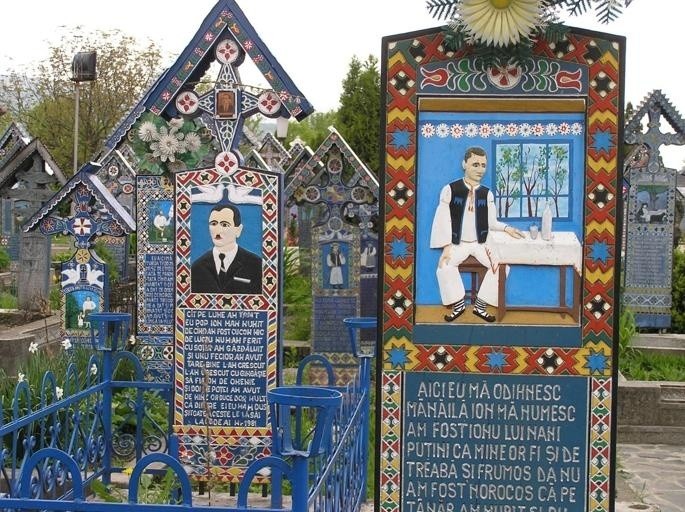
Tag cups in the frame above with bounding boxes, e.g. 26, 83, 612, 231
529, 226, 538, 240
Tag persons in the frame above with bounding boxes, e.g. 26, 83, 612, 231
218, 94, 235, 117
323, 240, 346, 292
190, 204, 262, 295
77, 310, 84, 330
154, 208, 172, 242
429, 146, 528, 323
80, 295, 97, 330
637, 201, 651, 224
631, 144, 649, 168
361, 241, 377, 267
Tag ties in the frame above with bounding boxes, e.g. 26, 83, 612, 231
218, 254, 225, 288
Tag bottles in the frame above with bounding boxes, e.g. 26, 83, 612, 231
541, 201, 552, 241
